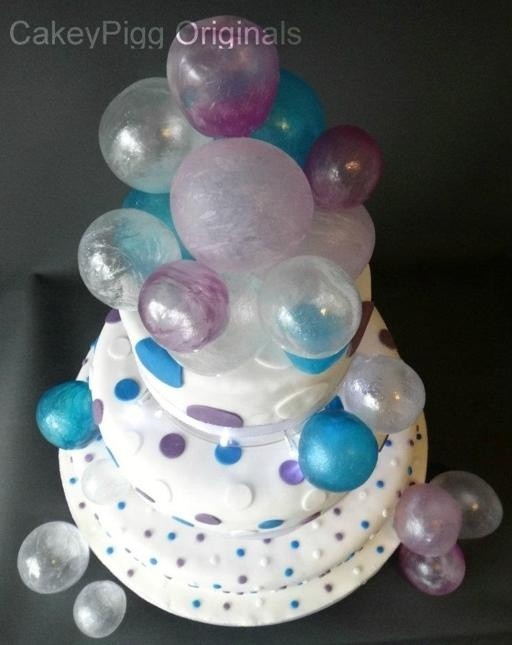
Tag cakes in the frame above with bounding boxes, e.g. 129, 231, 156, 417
59, 305, 428, 622
